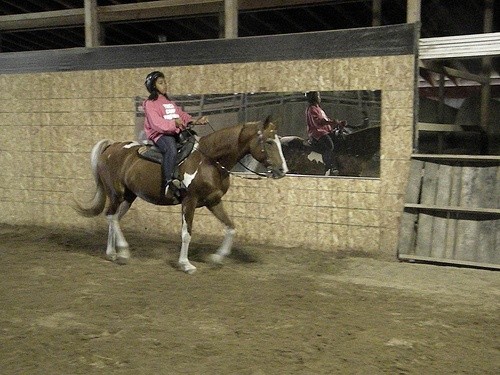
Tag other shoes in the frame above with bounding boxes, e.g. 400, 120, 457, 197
326, 162, 337, 175
167, 182, 186, 204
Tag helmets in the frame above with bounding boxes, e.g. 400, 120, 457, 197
304, 91, 316, 103
145, 71, 163, 92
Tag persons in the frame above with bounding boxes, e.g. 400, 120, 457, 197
302, 91, 350, 176
143, 70, 208, 200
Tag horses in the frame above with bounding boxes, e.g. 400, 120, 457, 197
245, 123, 380, 177
70, 113, 290, 275
